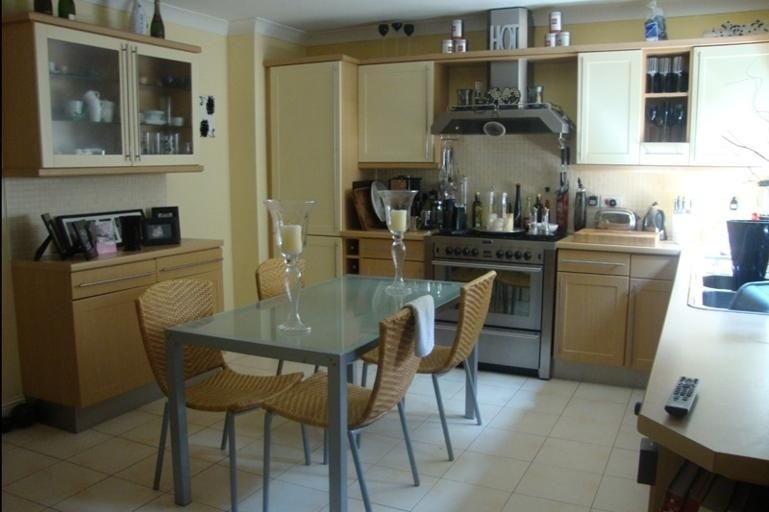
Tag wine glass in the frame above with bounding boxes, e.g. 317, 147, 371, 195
377, 189, 420, 297
262, 198, 318, 335
646, 55, 687, 142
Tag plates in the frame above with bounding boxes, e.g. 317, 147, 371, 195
145, 120, 166, 125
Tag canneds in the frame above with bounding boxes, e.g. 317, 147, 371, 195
543, 32, 555, 47
441, 38, 453, 54
453, 38, 466, 53
548, 11, 562, 33
556, 31, 570, 47
450, 18, 463, 39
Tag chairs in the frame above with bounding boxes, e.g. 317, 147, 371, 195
261, 294, 435, 510
361, 270, 497, 461
134, 280, 310, 490
255, 258, 322, 376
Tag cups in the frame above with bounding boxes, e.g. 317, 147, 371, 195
726, 220, 769, 290
64, 89, 114, 121
146, 110, 165, 119
173, 117, 184, 126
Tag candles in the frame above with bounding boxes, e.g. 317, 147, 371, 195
280, 223, 303, 254
390, 209, 407, 235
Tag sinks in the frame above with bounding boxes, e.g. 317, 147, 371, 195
689, 271, 769, 290
686, 290, 769, 316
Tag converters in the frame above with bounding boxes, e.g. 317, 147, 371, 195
588, 196, 598, 206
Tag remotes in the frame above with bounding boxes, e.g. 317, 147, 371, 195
664, 375, 700, 416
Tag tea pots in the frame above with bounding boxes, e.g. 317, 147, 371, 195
642, 202, 667, 241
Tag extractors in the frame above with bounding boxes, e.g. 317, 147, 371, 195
428, 9, 576, 135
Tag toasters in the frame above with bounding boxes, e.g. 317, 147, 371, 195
594, 208, 642, 231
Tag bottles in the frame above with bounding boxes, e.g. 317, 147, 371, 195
420, 183, 550, 234
128, 1, 165, 38
644, 2, 667, 41
573, 188, 587, 232
33, 0, 77, 22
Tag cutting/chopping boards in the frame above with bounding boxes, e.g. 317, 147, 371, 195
573, 227, 661, 247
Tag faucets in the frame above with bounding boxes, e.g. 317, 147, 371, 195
757, 178, 769, 188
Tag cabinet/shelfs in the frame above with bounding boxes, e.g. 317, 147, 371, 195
10, 238, 225, 432
264, 53, 358, 288
0, 12, 205, 177
577, 33, 768, 166
552, 228, 682, 389
358, 56, 449, 170
341, 228, 439, 278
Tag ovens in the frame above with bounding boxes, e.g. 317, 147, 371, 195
430, 235, 557, 331
